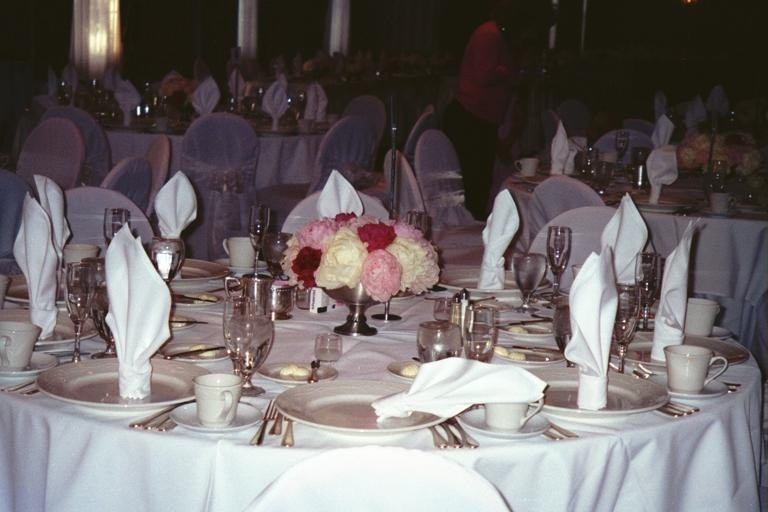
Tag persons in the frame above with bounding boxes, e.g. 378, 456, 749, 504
442, 9, 519, 220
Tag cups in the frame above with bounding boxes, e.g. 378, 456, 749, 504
514, 158, 540, 179
526, 132, 768, 216
56, 74, 316, 135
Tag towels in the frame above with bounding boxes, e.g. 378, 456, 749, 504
365, 352, 551, 422
556, 244, 619, 412
96, 221, 175, 401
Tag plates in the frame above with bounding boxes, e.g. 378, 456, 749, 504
0, 202, 750, 452
